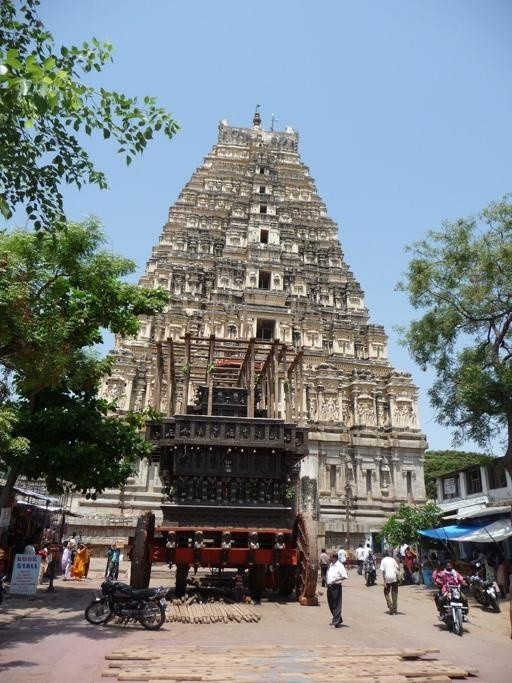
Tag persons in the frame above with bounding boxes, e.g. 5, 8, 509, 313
61, 531, 95, 580
324, 551, 348, 627
379, 549, 401, 616
402, 546, 441, 584
471, 549, 489, 564
106, 540, 121, 580
362, 544, 374, 561
431, 560, 444, 615
105, 545, 114, 578
23, 536, 36, 555
363, 550, 376, 579
434, 560, 470, 622
355, 543, 365, 574
318, 548, 330, 587
337, 545, 347, 566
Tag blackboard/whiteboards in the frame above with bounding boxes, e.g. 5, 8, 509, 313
10, 553, 42, 595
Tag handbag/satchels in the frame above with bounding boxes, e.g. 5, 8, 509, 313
396, 571, 404, 585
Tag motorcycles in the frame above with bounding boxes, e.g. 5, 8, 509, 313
85, 575, 170, 630
365, 558, 376, 586
463, 562, 501, 612
438, 574, 468, 634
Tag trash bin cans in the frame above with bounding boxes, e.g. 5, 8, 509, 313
423, 569, 431, 585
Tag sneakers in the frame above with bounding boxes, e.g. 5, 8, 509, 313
328, 619, 343, 629
